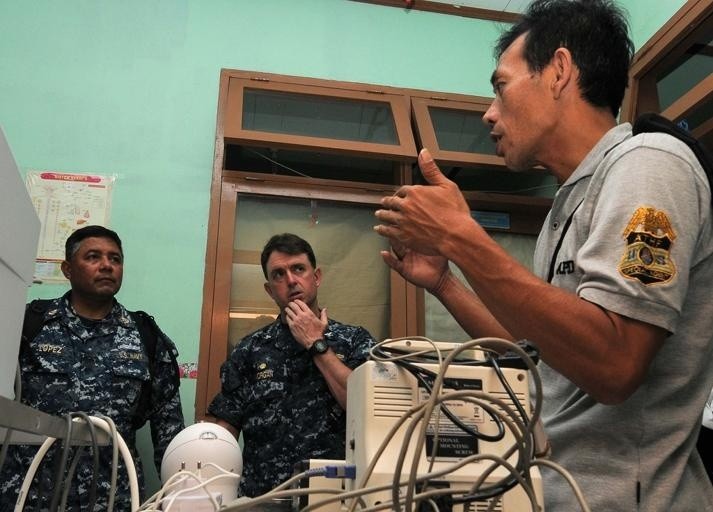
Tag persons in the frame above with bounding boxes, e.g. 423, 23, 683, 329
371, 1, 713, 512
205, 234, 383, 511
1, 225, 187, 512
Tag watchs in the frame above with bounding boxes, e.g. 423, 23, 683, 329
306, 338, 331, 360
